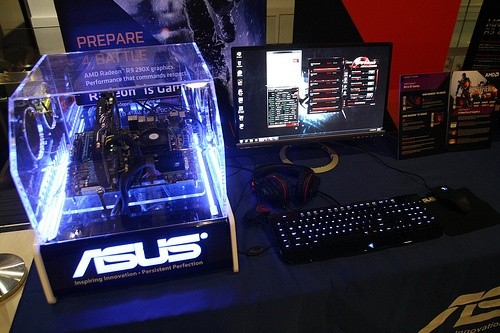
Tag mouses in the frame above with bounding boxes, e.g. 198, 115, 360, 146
430, 185, 471, 214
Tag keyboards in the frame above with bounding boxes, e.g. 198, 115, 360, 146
271, 193, 444, 265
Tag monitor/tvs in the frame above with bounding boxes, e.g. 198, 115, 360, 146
231, 42, 392, 149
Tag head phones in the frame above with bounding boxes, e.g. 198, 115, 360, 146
250, 163, 319, 204
